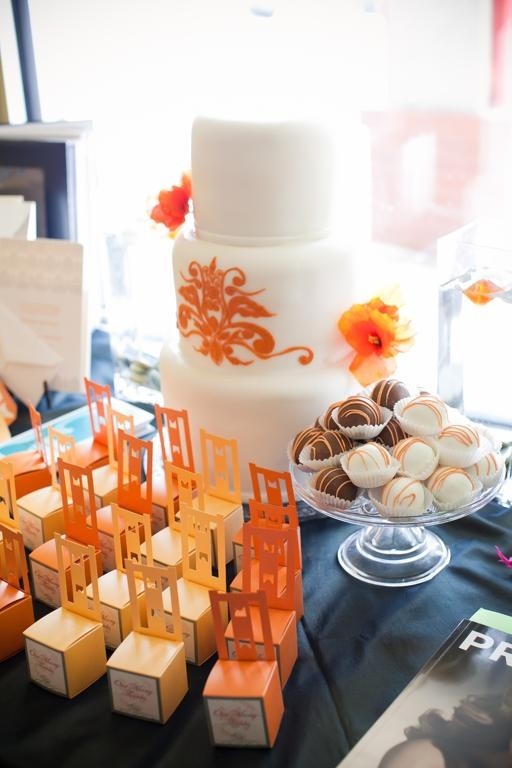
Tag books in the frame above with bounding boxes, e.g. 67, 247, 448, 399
336, 606, 512, 768
